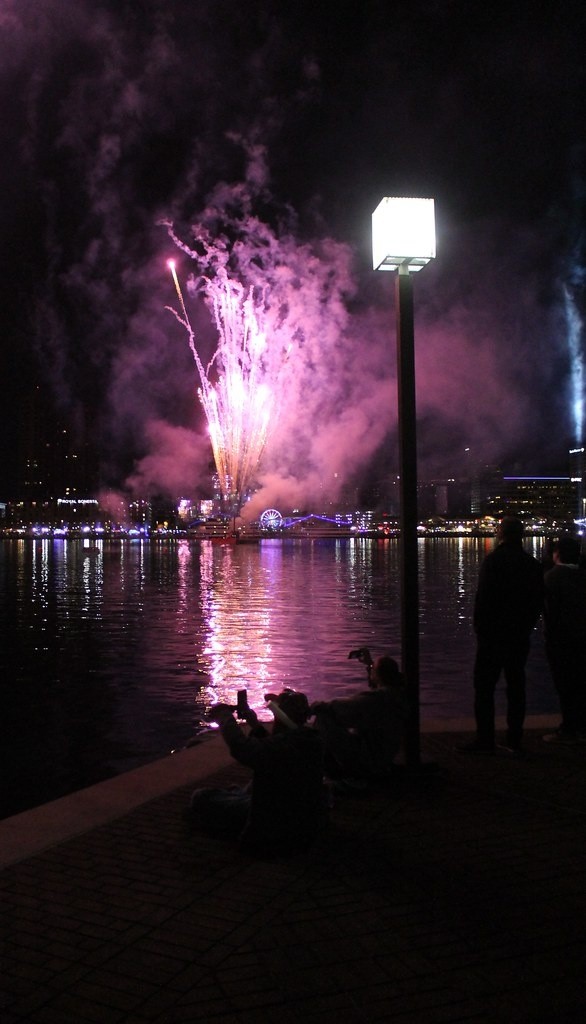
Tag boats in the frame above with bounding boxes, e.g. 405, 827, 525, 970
244, 524, 263, 543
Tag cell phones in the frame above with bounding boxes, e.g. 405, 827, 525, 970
347, 651, 364, 659
237, 690, 248, 720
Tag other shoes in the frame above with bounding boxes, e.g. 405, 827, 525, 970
575, 726, 586, 742
456, 740, 496, 753
497, 738, 520, 752
543, 727, 578, 744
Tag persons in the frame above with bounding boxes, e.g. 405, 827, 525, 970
459, 516, 544, 754
182, 689, 325, 859
542, 537, 586, 744
308, 647, 406, 799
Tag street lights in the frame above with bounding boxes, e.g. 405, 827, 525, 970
367, 195, 443, 768
